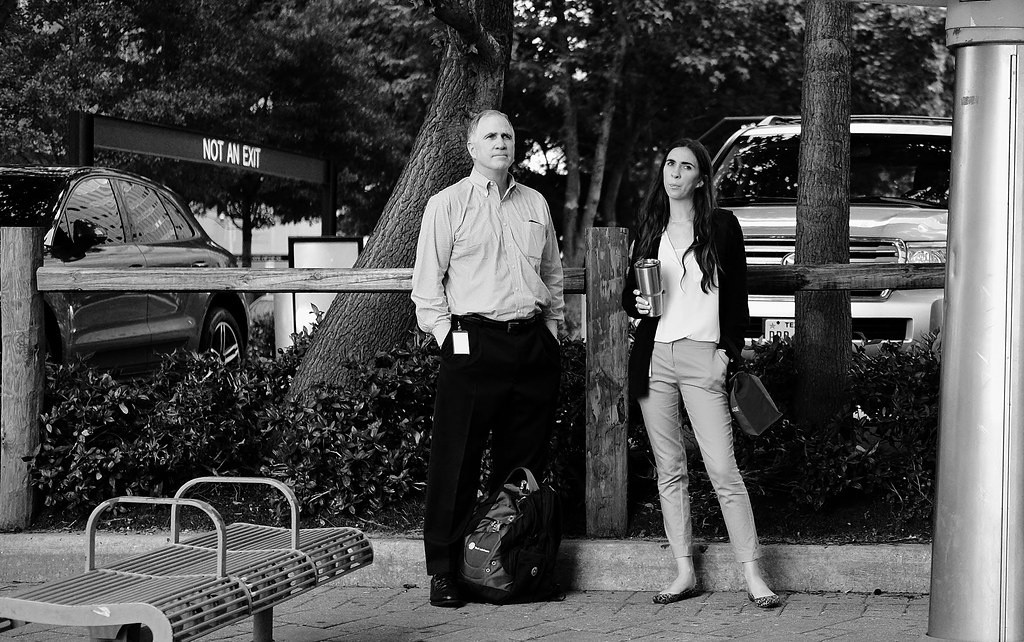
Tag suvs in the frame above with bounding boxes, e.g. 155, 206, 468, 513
709, 112, 955, 363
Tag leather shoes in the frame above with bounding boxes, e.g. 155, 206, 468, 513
430, 575, 461, 605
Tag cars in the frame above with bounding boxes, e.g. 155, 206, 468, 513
0, 163, 252, 378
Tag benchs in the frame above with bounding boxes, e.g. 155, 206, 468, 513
0, 476, 372, 642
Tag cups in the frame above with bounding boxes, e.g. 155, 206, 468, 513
634, 259, 663, 317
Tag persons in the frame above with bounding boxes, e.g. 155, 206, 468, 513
410, 110, 567, 607
618, 138, 781, 609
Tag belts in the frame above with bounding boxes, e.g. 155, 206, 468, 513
452, 315, 542, 334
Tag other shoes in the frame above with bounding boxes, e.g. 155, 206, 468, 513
652, 579, 697, 604
748, 588, 781, 608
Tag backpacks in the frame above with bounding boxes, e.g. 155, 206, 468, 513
457, 466, 562, 605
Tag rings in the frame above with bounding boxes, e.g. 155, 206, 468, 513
634, 303, 639, 309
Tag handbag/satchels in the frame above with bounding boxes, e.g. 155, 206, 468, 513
728, 371, 783, 437
